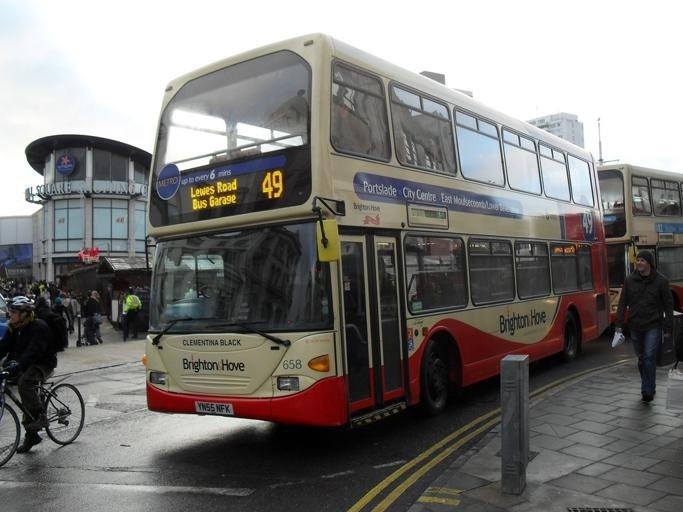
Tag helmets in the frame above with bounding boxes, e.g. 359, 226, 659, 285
5, 296, 35, 312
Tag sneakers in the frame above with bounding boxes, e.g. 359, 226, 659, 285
17, 435, 42, 452
29, 414, 49, 430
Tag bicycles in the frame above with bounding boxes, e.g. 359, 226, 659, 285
0, 369, 83, 464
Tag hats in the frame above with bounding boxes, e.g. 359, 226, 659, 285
637, 251, 654, 266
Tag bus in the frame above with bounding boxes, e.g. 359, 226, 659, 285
140, 32, 611, 433
597, 162, 682, 327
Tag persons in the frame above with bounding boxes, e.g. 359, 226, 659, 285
613, 250, 674, 403
0, 276, 142, 454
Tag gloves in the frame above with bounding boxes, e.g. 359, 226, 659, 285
4, 364, 19, 375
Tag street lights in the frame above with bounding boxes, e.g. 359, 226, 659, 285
594, 117, 603, 166
